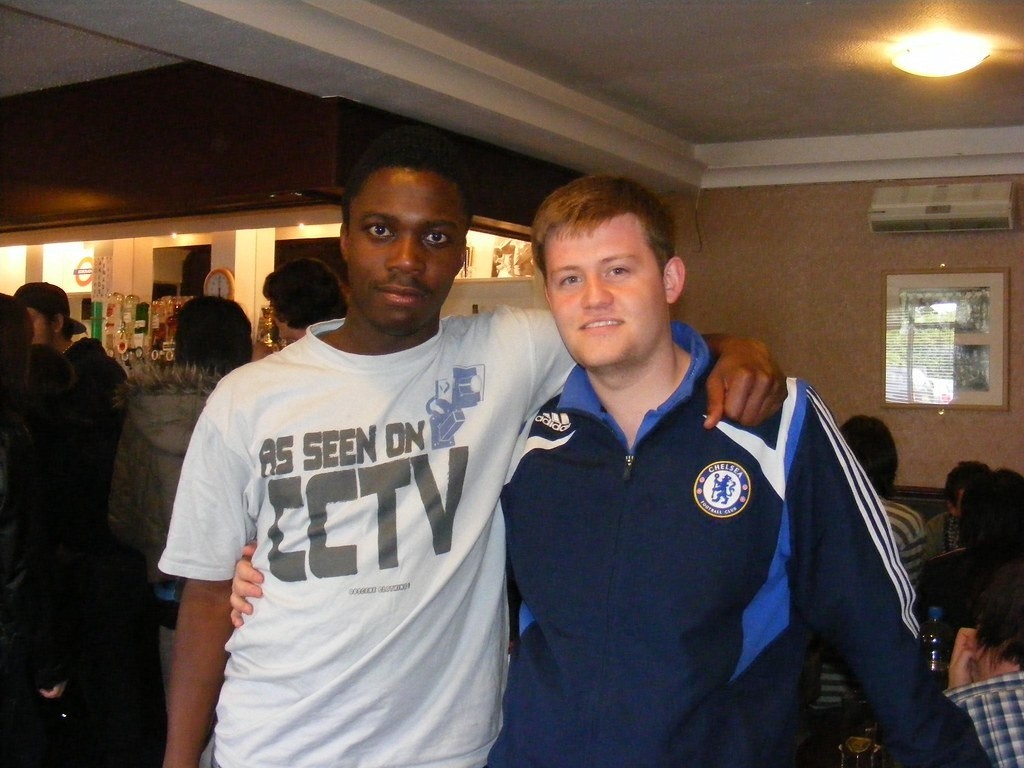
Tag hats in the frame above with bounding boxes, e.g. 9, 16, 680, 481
14, 282, 87, 334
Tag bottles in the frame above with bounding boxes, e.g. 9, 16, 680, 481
106, 293, 149, 349
91, 302, 102, 339
150, 296, 195, 342
922, 607, 949, 681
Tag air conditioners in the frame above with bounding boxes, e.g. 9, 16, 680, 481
867, 182, 1015, 234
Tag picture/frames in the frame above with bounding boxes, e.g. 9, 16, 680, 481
880, 267, 1010, 412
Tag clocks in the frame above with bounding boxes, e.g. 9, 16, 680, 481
203, 268, 235, 301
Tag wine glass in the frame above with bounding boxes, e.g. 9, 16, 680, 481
260, 307, 276, 344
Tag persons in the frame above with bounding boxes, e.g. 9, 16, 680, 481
159, 139, 789, 768
0, 255, 1023, 768
227, 172, 990, 767
